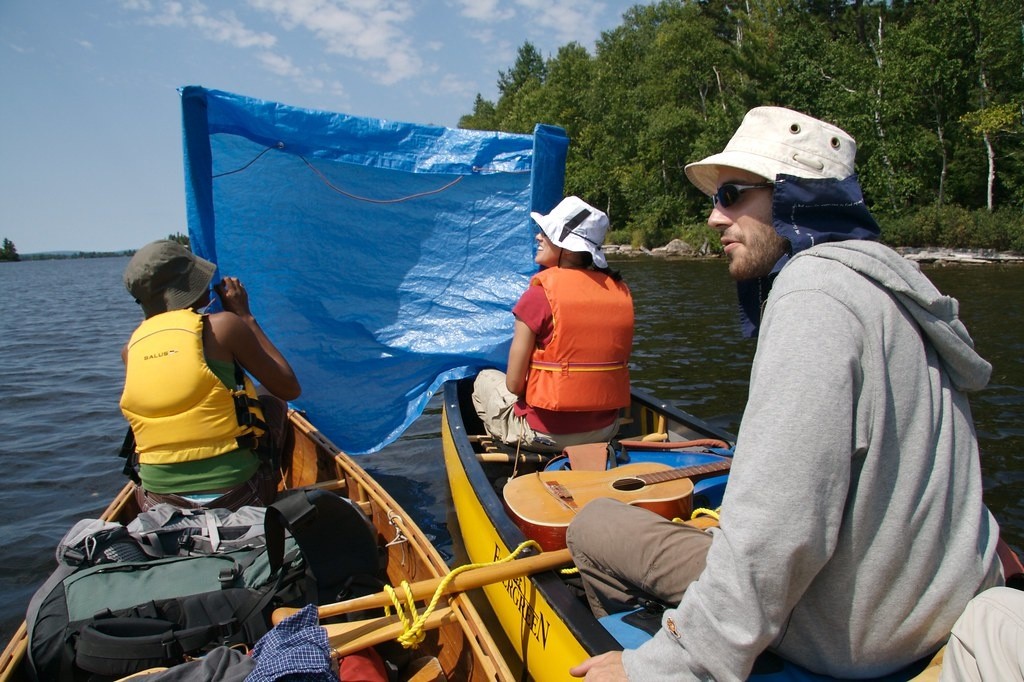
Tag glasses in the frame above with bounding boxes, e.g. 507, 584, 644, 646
712, 182, 774, 210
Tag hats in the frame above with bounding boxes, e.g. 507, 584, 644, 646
123, 239, 217, 320
684, 106, 856, 197
531, 195, 610, 268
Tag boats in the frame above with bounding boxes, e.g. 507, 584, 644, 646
443, 374, 1024, 682
0, 390, 514, 681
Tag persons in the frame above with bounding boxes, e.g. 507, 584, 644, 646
118, 240, 301, 518
470, 195, 635, 452
565, 106, 1024, 682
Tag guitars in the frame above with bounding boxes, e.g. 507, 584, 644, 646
501, 458, 733, 554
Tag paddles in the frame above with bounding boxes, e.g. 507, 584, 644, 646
268, 507, 726, 630
